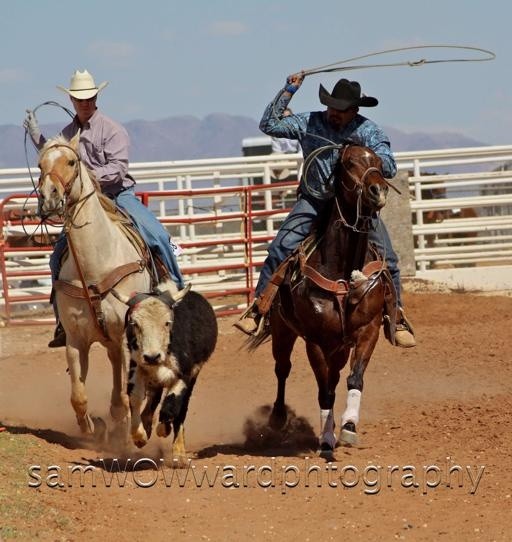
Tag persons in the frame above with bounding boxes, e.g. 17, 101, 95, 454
231, 69, 419, 348
23, 69, 184, 349
270, 108, 303, 155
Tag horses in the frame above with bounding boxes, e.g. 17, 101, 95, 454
235, 136, 389, 464
409, 169, 450, 270
32, 125, 157, 448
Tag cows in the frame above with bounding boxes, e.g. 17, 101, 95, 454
448, 205, 480, 269
106, 281, 221, 461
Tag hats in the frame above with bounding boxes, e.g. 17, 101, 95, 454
58, 71, 108, 100
320, 79, 378, 110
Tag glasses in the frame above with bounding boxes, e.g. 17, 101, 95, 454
71, 97, 96, 101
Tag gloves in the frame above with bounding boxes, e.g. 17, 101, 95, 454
23, 109, 45, 144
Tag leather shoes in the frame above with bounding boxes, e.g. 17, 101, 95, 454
49, 330, 66, 347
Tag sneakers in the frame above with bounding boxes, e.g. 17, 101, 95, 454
395, 323, 416, 348
235, 318, 266, 336
